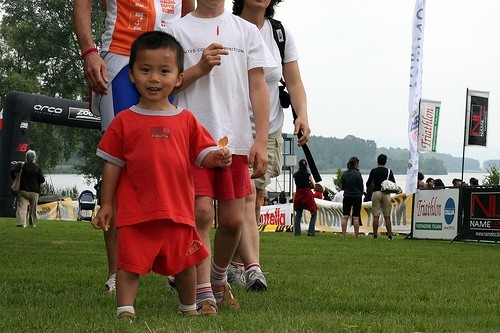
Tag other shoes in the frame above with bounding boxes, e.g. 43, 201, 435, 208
196, 299, 218, 315
214, 283, 240, 310
104, 274, 115, 291
116, 312, 137, 322
308, 233, 313, 236
17, 224, 26, 228
168, 276, 176, 288
388, 236, 392, 240
179, 309, 199, 318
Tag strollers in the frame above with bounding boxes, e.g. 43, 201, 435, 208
77, 189, 96, 221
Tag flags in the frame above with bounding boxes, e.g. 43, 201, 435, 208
406, 0, 424, 196
420, 99, 441, 153
467, 90, 490, 147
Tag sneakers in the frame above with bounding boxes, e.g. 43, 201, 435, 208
245, 270, 267, 291
228, 266, 247, 287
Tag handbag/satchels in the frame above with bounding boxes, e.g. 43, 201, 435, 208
380, 168, 399, 193
11, 161, 25, 191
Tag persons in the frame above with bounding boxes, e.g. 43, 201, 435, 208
9, 150, 45, 228
333, 185, 344, 203
90, 28, 232, 323
365, 154, 395, 241
74, 0, 196, 295
292, 158, 318, 237
417, 172, 479, 190
363, 189, 372, 201
166, 0, 279, 315
340, 156, 364, 237
227, 0, 311, 292
261, 190, 295, 206
312, 183, 332, 201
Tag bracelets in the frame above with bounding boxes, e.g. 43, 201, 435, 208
81, 48, 98, 60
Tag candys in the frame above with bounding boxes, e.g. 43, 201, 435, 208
217, 25, 220, 43
219, 136, 228, 151
